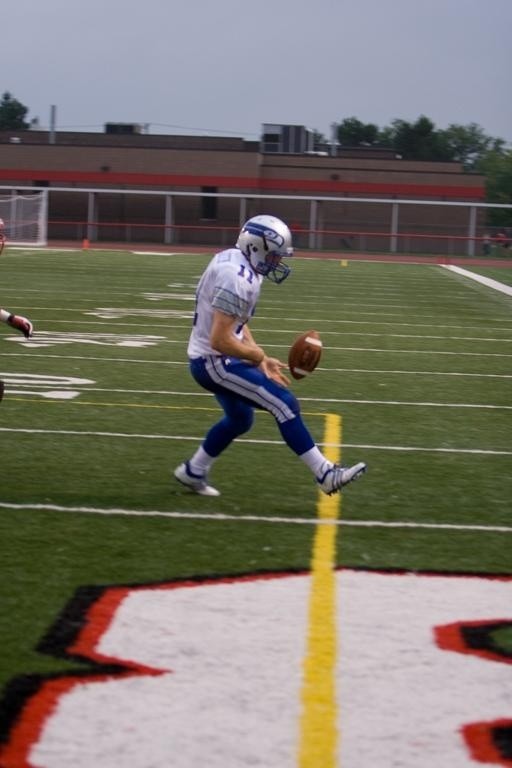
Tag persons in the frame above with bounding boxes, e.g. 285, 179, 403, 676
495, 230, 507, 247
0, 216, 33, 403
481, 229, 492, 256
173, 213, 367, 498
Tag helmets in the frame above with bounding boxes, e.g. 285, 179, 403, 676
235, 214, 294, 284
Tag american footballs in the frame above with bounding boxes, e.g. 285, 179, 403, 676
289, 329, 321, 380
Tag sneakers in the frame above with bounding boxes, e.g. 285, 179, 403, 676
312, 461, 367, 495
174, 461, 221, 496
